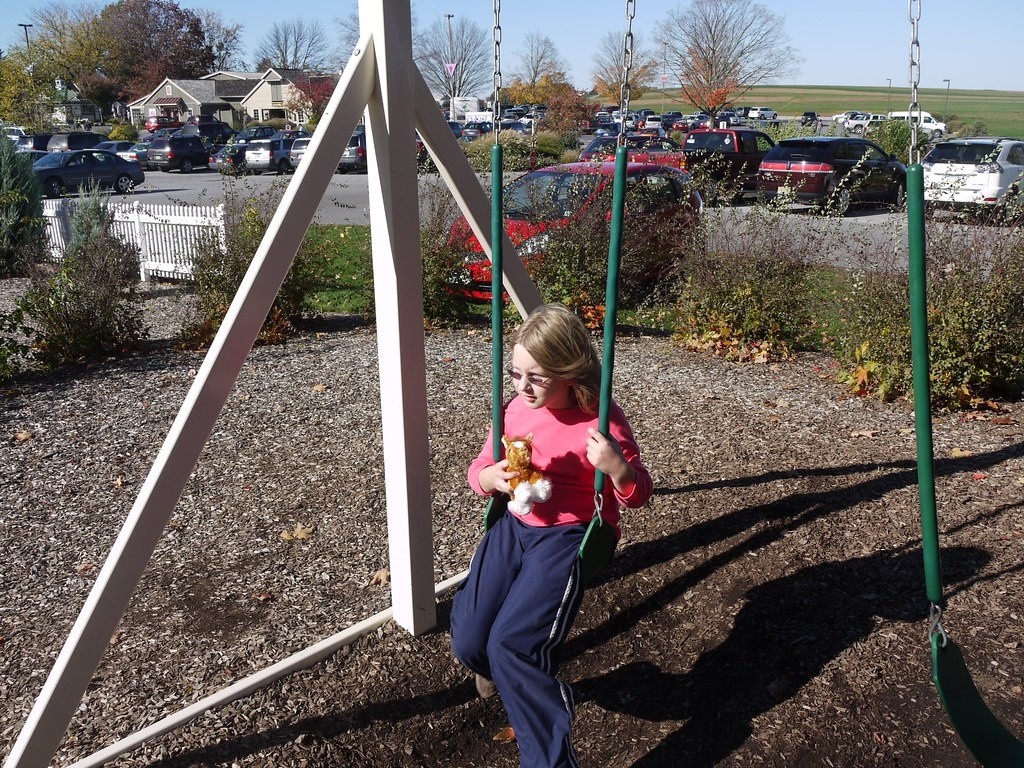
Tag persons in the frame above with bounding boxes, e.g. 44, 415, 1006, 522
447, 303, 654, 768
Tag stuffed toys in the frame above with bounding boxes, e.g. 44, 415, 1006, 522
501, 432, 552, 516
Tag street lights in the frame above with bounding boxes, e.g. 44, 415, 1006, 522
887, 79, 891, 114
943, 79, 950, 117
17, 24, 34, 67
661, 42, 668, 114
444, 14, 455, 97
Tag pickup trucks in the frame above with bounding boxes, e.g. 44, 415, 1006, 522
627, 129, 776, 201
844, 114, 887, 134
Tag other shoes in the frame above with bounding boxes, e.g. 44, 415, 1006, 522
476, 674, 498, 699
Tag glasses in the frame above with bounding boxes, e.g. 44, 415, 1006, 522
506, 367, 551, 386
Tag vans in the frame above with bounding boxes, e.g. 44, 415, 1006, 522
887, 111, 949, 137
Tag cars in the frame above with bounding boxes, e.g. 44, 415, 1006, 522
16, 150, 47, 162
832, 112, 872, 125
436, 160, 704, 307
271, 129, 310, 139
116, 143, 149, 169
578, 131, 683, 164
142, 128, 180, 140
4, 127, 36, 142
864, 120, 888, 136
208, 144, 251, 174
30, 148, 145, 197
233, 125, 278, 143
93, 140, 135, 161
415, 101, 742, 164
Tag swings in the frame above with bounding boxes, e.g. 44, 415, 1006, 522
906, 1, 1023, 768
480, 0, 635, 585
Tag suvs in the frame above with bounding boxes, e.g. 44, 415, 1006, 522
801, 112, 820, 126
144, 116, 186, 133
921, 137, 1024, 225
337, 130, 367, 174
736, 107, 752, 118
46, 131, 112, 152
756, 137, 907, 214
16, 133, 53, 151
146, 134, 225, 174
177, 122, 241, 143
748, 107, 778, 119
245, 139, 295, 175
187, 115, 220, 125
291, 137, 311, 170
